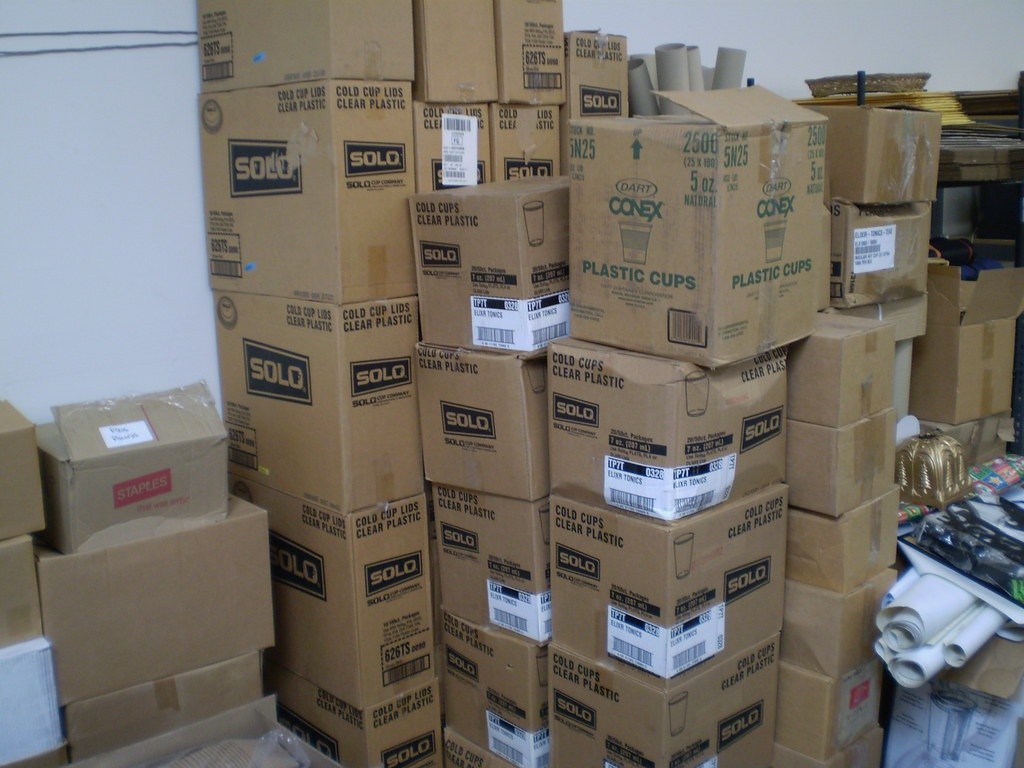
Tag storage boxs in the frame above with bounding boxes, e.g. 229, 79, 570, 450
2, 399, 48, 537
0, 536, 48, 651
35, 378, 229, 556
0, 640, 69, 765
34, 493, 273, 708
197, 0, 1024, 768
2, 749, 69, 768
69, 692, 350, 768
69, 649, 266, 761
